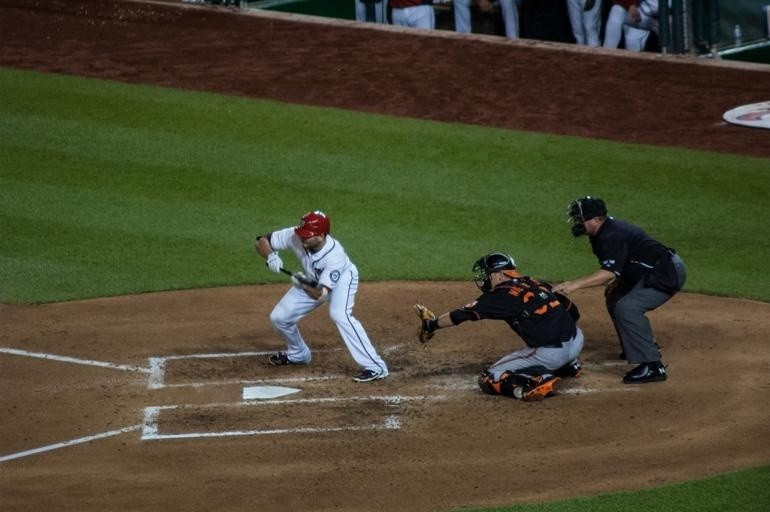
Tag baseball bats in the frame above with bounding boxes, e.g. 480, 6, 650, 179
280, 269, 332, 295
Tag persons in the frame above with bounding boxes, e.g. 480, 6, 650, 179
390, 0, 660, 53
413, 251, 585, 402
254, 210, 389, 382
550, 194, 687, 383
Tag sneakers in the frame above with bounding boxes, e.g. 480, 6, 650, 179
525, 376, 561, 400
269, 352, 291, 367
565, 361, 584, 378
353, 370, 390, 384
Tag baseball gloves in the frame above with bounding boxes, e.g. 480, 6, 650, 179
412, 305, 436, 343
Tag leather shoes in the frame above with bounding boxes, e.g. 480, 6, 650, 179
623, 364, 668, 384
619, 341, 664, 360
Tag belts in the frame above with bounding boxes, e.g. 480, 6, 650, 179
540, 328, 578, 348
655, 248, 677, 270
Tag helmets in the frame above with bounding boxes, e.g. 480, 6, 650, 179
294, 211, 330, 238
474, 253, 521, 295
568, 197, 607, 237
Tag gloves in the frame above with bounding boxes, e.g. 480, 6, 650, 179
267, 251, 283, 274
291, 271, 310, 291
